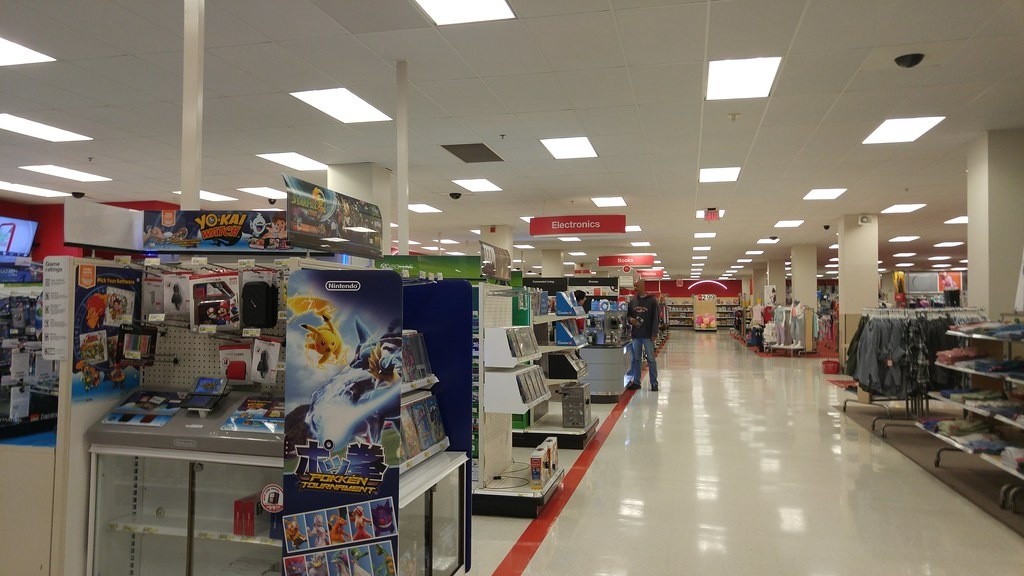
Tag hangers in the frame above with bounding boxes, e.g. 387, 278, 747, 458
862, 294, 989, 326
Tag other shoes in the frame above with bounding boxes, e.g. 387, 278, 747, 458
629, 383, 641, 390
651, 384, 658, 391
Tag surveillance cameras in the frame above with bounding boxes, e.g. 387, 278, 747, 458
449, 193, 461, 200
268, 199, 276, 204
72, 192, 84, 198
824, 225, 830, 230
894, 54, 924, 68
770, 237, 777, 240
516, 268, 542, 275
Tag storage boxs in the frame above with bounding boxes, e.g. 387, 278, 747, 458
531, 445, 551, 490
540, 437, 560, 478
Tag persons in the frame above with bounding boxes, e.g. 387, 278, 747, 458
753, 304, 766, 353
574, 290, 587, 305
626, 279, 660, 391
774, 305, 786, 347
789, 299, 804, 347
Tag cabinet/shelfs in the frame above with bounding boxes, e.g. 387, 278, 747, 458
53, 251, 753, 576
914, 313, 1023, 514
